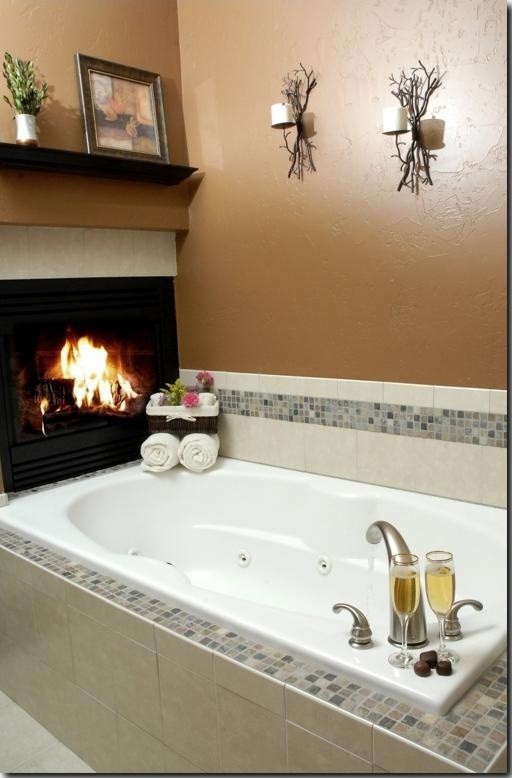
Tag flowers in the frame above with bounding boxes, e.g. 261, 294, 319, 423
160, 370, 214, 404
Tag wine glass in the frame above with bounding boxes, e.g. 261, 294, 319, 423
388, 551, 462, 669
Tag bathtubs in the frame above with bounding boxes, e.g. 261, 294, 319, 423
0, 456, 510, 714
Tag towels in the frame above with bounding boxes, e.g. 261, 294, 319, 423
139, 432, 219, 472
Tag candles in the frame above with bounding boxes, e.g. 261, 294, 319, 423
270, 101, 293, 124
381, 105, 407, 133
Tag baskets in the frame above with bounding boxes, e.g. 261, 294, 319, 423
145, 392, 219, 434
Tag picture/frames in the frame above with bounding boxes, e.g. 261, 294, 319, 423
73, 51, 172, 164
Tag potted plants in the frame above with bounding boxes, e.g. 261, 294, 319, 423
1, 51, 50, 146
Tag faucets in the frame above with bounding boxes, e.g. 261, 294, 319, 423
367, 521, 431, 647
